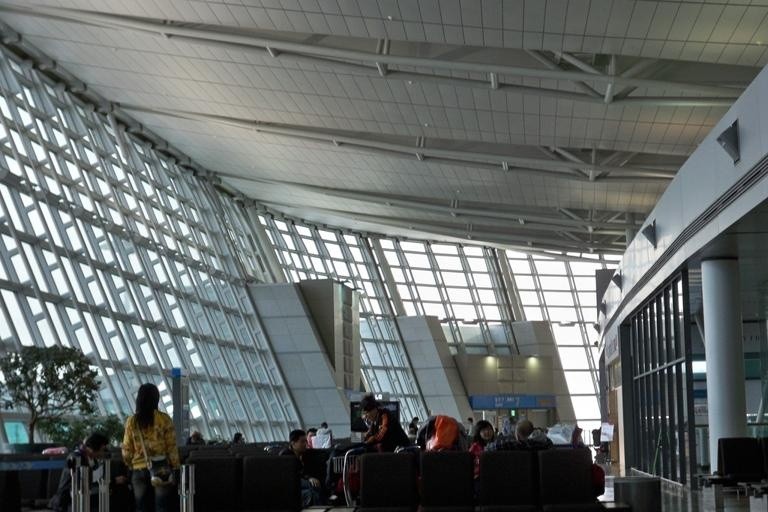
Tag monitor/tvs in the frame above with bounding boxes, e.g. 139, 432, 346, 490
351, 401, 399, 432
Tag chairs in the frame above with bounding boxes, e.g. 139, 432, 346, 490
715, 437, 768, 510
179, 443, 606, 512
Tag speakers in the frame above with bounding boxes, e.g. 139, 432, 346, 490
594, 341, 598, 347
641, 218, 657, 248
599, 300, 606, 319
594, 323, 600, 334
612, 269, 622, 293
716, 118, 740, 163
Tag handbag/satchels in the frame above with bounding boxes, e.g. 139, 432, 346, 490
148, 458, 175, 486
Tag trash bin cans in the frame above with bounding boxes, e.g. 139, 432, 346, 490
614, 477, 661, 511
180, 464, 196, 512
69, 456, 111, 512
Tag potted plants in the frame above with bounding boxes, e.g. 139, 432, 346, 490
2, 346, 99, 455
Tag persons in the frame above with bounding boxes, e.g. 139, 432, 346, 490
185, 431, 206, 446
224, 432, 248, 448
409, 416, 419, 435
47, 431, 126, 512
121, 383, 179, 512
465, 413, 552, 492
278, 422, 332, 512
360, 393, 411, 453
